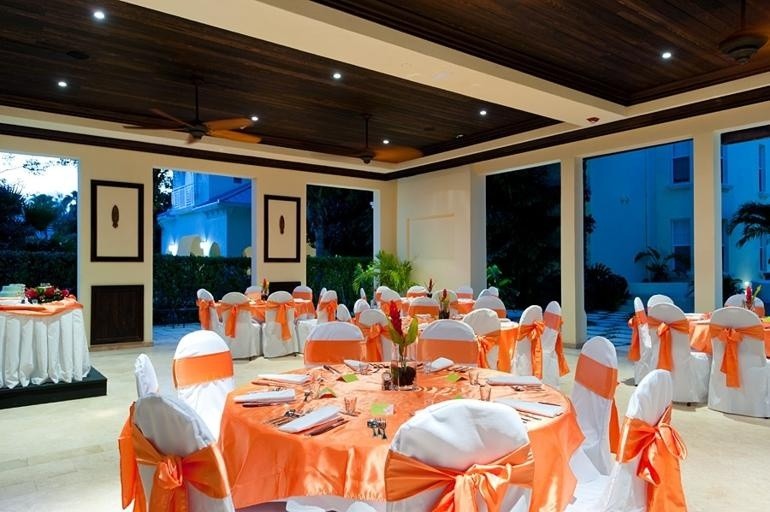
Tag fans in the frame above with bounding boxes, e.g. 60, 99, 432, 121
123, 72, 261, 148
324, 111, 422, 166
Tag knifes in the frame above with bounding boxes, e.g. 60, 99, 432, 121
309, 419, 349, 436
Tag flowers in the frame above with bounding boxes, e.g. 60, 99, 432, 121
20, 284, 69, 304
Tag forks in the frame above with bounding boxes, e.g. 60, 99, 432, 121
379, 419, 388, 439
266, 408, 312, 426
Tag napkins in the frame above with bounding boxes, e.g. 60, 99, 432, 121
497, 397, 563, 418
278, 404, 341, 433
234, 389, 296, 405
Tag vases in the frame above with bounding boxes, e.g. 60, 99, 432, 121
390, 361, 417, 391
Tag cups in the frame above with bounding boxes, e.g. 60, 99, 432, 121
310, 358, 492, 415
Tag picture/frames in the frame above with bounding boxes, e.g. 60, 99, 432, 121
263, 192, 301, 263
90, 178, 144, 262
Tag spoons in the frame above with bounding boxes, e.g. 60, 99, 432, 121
368, 416, 379, 436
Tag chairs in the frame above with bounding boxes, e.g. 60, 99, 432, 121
382, 400, 535, 511
689, 283, 770, 421
631, 293, 712, 408
198, 284, 570, 387
120, 396, 233, 512
566, 368, 687, 511
172, 330, 233, 440
562, 335, 622, 480
134, 353, 159, 400
